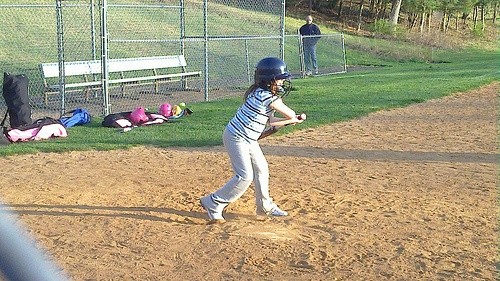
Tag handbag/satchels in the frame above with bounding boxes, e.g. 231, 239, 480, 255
0, 72, 32, 126
3, 117, 68, 143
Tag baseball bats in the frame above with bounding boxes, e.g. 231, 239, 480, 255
257, 112, 307, 140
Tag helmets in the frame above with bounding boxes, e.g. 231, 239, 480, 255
159, 103, 172, 117
129, 108, 149, 123
254, 58, 293, 83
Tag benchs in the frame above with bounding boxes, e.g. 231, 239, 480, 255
38, 54, 202, 106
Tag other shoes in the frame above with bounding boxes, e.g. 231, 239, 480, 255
306, 70, 312, 75
315, 71, 318, 74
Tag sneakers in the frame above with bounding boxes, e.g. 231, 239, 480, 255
200, 194, 226, 222
256, 206, 288, 216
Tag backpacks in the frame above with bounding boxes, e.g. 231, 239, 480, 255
60, 107, 91, 128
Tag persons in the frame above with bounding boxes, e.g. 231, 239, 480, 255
199, 56, 304, 221
300, 14, 321, 75
